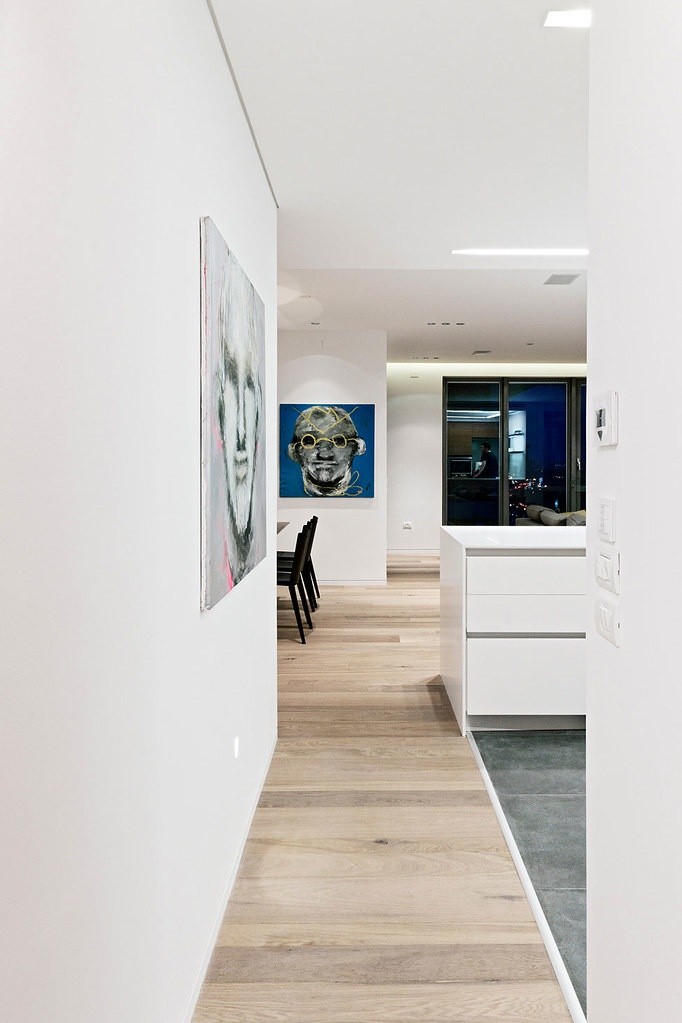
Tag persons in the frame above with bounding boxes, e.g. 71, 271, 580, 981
469, 442, 499, 478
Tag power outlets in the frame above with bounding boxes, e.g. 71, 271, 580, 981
403, 522, 411, 529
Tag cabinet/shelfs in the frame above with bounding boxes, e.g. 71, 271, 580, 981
441, 526, 586, 738
448, 422, 499, 456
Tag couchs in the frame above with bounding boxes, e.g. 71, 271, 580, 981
515, 505, 586, 526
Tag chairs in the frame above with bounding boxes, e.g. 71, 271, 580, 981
277, 516, 320, 644
